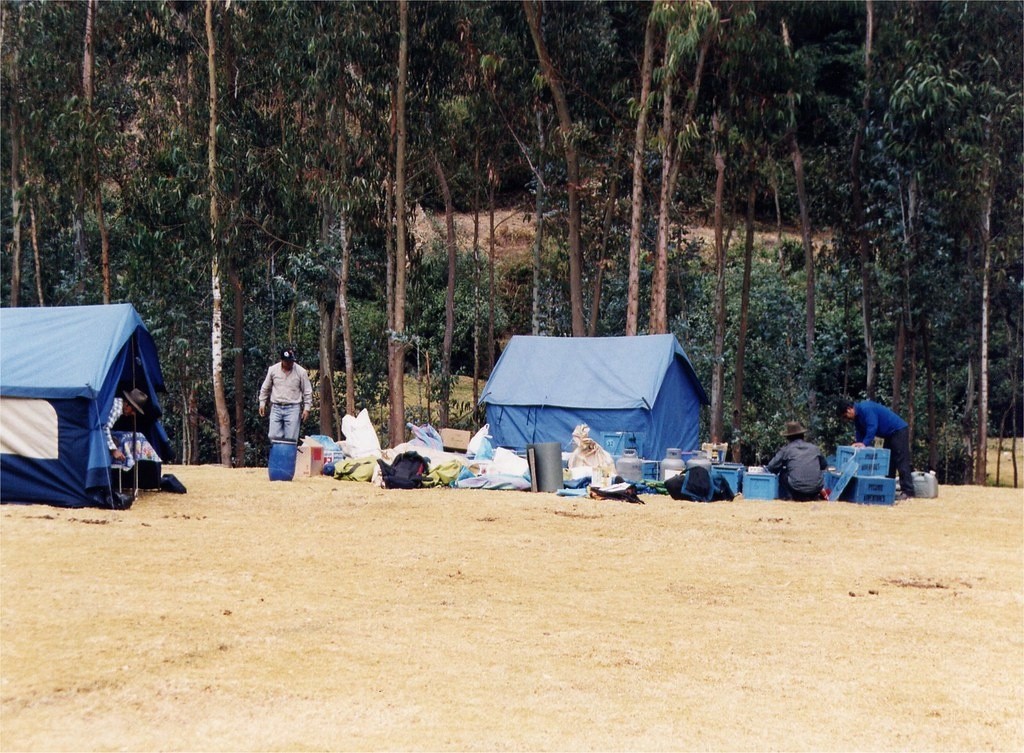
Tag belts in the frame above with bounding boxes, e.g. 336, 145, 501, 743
275, 403, 293, 406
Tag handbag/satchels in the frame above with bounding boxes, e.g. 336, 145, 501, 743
662, 466, 741, 505
336, 456, 378, 484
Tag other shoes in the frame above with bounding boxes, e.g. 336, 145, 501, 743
895, 493, 915, 500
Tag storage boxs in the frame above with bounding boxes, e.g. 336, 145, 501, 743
438, 428, 471, 450
294, 436, 344, 477
823, 446, 896, 505
600, 430, 780, 500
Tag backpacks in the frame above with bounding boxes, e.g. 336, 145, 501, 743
377, 450, 433, 493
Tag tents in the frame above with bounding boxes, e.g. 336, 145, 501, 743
475, 330, 712, 481
0, 303, 176, 512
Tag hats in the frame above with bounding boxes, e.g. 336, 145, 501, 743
124, 389, 148, 415
780, 422, 808, 437
281, 349, 297, 363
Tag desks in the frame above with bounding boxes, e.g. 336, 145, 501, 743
107, 431, 163, 501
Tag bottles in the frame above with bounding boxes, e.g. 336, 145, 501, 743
711, 442, 718, 463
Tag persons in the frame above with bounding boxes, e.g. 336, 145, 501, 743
835, 400, 916, 501
103, 387, 148, 462
767, 421, 828, 502
258, 346, 313, 442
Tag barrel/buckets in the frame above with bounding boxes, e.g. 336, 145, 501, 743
526, 442, 563, 493
268, 438, 297, 481
